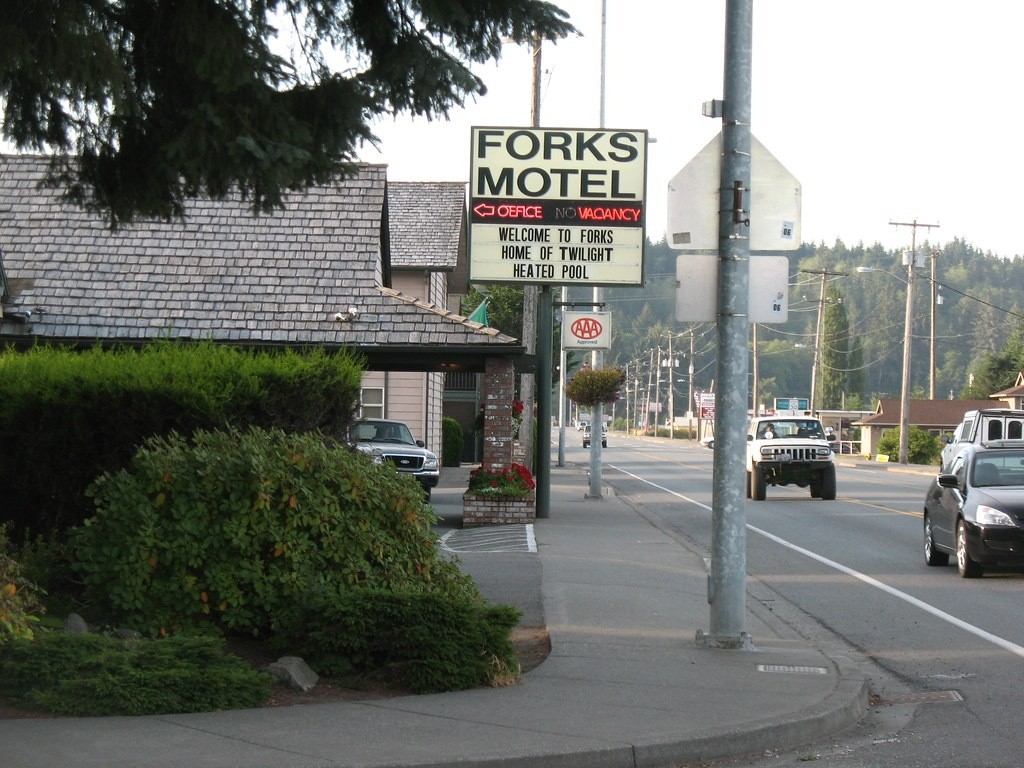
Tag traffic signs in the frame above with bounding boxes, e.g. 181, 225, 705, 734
464, 123, 650, 290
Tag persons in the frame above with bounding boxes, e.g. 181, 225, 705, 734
765, 424, 778, 437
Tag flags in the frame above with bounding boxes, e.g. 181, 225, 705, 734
470, 303, 488, 327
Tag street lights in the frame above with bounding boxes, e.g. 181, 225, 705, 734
647, 383, 660, 438
855, 264, 916, 465
625, 379, 639, 431
639, 387, 652, 435
676, 378, 695, 440
794, 342, 820, 417
658, 379, 674, 439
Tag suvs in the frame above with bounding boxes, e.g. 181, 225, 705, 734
345, 418, 441, 504
746, 414, 837, 503
581, 424, 608, 449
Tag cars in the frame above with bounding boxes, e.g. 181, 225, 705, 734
699, 437, 714, 450
921, 440, 1024, 579
577, 420, 588, 431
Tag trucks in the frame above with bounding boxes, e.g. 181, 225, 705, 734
937, 406, 1024, 475
